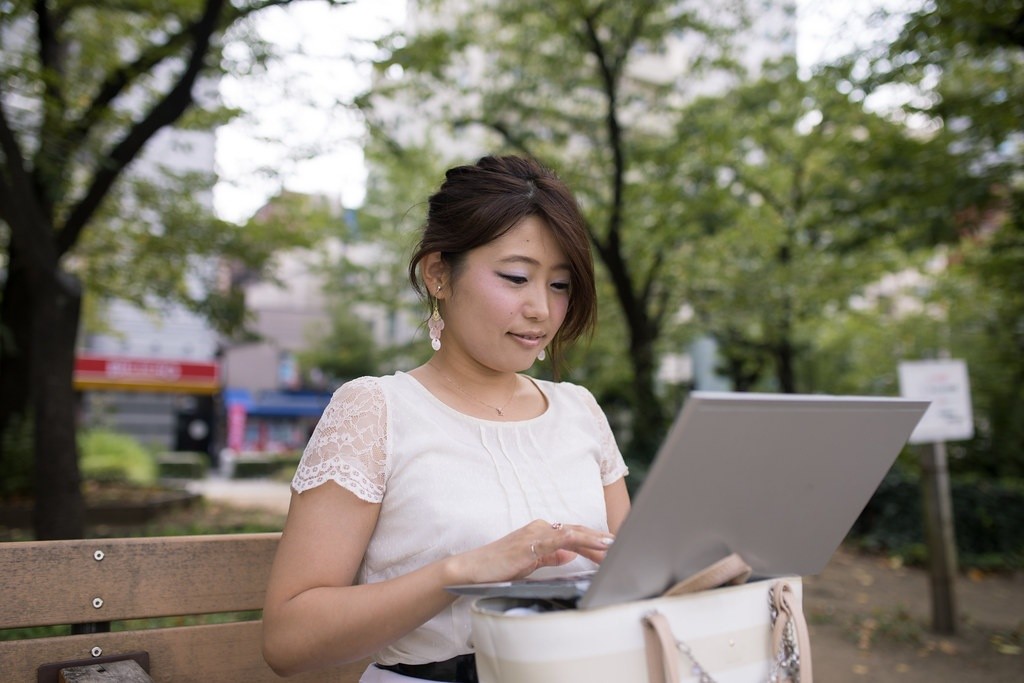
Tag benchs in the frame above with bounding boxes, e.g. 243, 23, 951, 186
0, 534, 379, 682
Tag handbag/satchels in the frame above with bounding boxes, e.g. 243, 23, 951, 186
473, 575, 812, 683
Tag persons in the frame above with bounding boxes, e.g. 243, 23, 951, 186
262, 156, 631, 683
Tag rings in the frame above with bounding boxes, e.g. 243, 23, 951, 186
531, 540, 544, 559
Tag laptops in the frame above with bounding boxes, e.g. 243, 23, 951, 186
443, 390, 932, 609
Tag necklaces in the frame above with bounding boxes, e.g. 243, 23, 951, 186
427, 360, 517, 416
